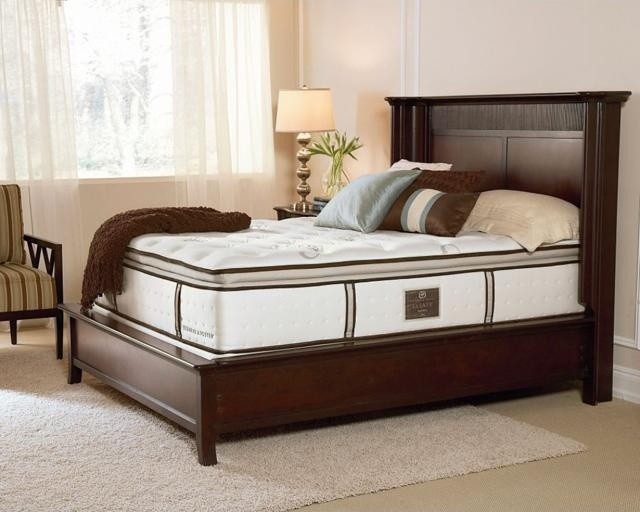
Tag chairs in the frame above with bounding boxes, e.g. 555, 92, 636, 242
0, 184, 64, 360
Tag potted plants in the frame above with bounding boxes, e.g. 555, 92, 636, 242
305, 129, 363, 198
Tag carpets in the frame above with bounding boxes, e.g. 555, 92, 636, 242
0, 346, 588, 511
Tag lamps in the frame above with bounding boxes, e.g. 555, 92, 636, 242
274, 86, 336, 211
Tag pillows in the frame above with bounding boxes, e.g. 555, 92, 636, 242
455, 189, 581, 253
389, 160, 453, 172
313, 169, 423, 233
412, 169, 480, 192
376, 182, 481, 237
0, 184, 26, 265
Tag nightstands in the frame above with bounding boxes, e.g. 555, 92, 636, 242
272, 206, 320, 220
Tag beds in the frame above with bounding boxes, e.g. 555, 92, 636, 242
56, 91, 633, 466
